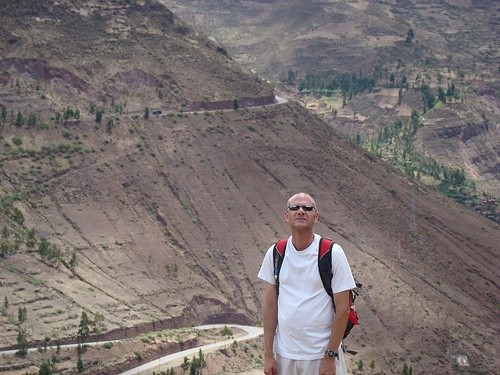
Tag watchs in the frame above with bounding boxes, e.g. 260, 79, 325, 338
324, 349, 338, 357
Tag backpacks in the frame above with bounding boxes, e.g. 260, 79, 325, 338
272, 237, 360, 344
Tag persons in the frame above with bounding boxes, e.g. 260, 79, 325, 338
256, 192, 357, 375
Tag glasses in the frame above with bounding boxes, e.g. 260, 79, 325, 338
286, 204, 316, 212
